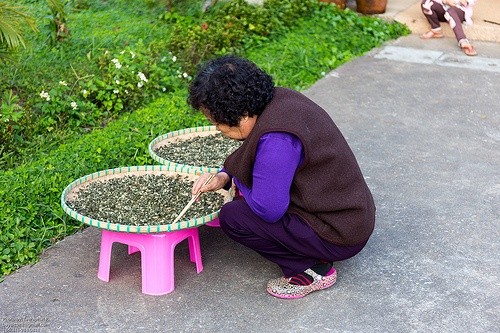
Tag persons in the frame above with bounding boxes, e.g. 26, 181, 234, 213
421, 0, 476, 57
187, 55, 375, 300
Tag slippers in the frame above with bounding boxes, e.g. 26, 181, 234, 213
267, 269, 337, 299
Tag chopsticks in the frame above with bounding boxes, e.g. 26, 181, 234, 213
172, 175, 215, 224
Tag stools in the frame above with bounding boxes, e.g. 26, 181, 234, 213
97, 228, 203, 295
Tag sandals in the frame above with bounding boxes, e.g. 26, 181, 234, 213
460, 45, 477, 56
421, 29, 443, 39
